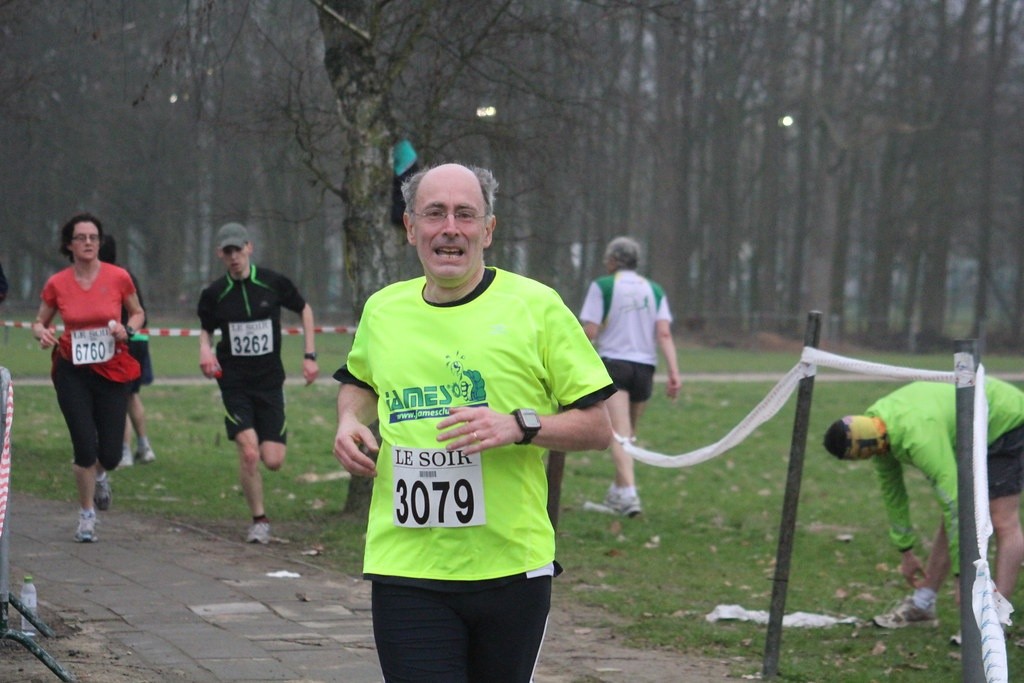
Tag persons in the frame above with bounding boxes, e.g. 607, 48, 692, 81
578, 238, 682, 516
823, 371, 1024, 648
34, 214, 144, 543
97, 235, 156, 466
332, 163, 617, 683
198, 222, 319, 544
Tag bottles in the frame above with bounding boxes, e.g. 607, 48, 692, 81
21, 577, 36, 636
214, 366, 222, 379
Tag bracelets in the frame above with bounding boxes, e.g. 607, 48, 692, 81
898, 545, 914, 552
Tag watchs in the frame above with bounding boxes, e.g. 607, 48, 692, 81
508, 407, 542, 445
305, 352, 317, 360
124, 324, 134, 342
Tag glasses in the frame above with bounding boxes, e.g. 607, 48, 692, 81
71, 235, 101, 244
412, 208, 486, 226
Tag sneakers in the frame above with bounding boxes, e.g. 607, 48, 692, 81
94, 473, 112, 510
603, 485, 640, 516
873, 596, 938, 629
950, 631, 961, 646
134, 445, 155, 462
74, 510, 98, 542
246, 521, 270, 544
118, 450, 133, 468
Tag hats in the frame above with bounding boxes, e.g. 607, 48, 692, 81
215, 222, 248, 252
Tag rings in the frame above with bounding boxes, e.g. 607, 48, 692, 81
473, 431, 478, 439
